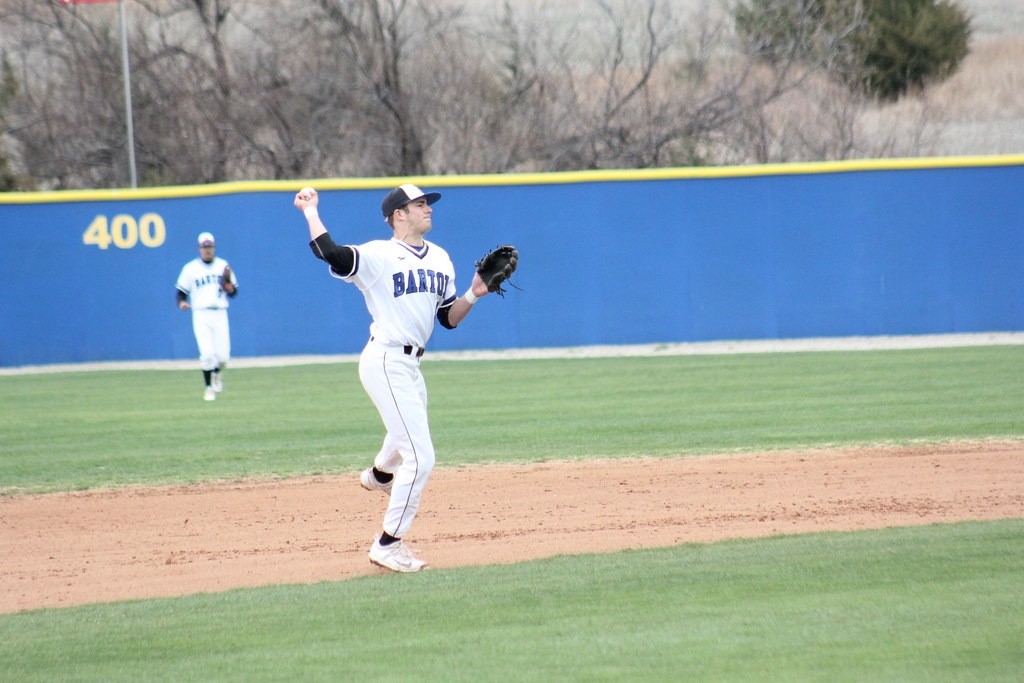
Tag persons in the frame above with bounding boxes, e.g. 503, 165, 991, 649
174, 231, 238, 402
293, 185, 519, 573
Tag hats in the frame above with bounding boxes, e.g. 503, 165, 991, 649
382, 184, 441, 223
199, 232, 214, 247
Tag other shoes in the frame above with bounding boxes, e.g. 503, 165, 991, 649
210, 371, 223, 393
203, 385, 215, 401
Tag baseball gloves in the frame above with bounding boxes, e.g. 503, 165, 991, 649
222, 265, 231, 286
475, 244, 519, 292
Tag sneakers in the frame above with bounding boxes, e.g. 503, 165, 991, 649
360, 466, 395, 496
369, 538, 425, 572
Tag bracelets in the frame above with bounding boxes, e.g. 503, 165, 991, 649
303, 206, 319, 221
464, 287, 480, 305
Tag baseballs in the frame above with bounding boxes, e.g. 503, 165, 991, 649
301, 187, 315, 199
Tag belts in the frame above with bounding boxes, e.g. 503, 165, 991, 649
371, 336, 425, 357
208, 307, 218, 310
